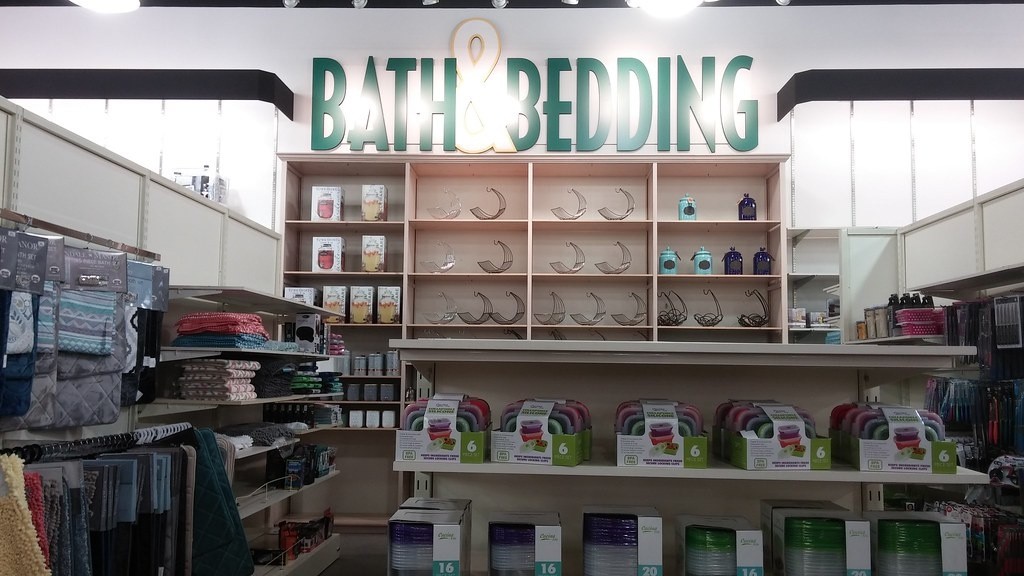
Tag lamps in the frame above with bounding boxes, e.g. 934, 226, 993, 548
490, 0, 508, 9
422, 0, 440, 6
351, 0, 368, 10
283, 0, 301, 9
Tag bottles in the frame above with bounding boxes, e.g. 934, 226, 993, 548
282, 186, 395, 324
889, 292, 934, 336
199, 164, 210, 197
660, 194, 772, 274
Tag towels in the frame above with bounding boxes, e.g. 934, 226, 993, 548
176, 358, 300, 402
169, 312, 299, 353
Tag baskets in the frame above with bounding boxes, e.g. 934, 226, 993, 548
896, 308, 945, 335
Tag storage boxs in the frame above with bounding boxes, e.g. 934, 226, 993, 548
395, 393, 957, 475
361, 235, 388, 273
310, 186, 345, 223
361, 184, 388, 221
312, 236, 346, 273
284, 285, 402, 324
388, 497, 968, 576
894, 308, 947, 336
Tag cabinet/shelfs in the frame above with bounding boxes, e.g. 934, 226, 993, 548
126, 150, 989, 574
